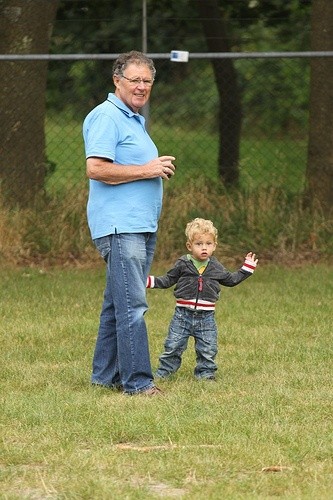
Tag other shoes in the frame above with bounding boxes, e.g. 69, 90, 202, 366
143, 385, 163, 397
206, 376, 215, 381
111, 382, 123, 392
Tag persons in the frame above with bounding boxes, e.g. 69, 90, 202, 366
83, 51, 176, 395
147, 218, 258, 380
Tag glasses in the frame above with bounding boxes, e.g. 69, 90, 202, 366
120, 74, 153, 87
